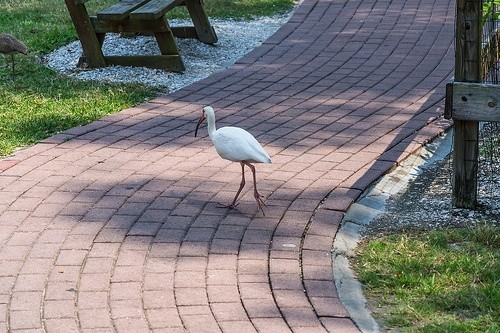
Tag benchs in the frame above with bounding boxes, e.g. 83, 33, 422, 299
63, 0, 219, 73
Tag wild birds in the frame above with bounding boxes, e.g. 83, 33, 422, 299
195, 106, 272, 217
